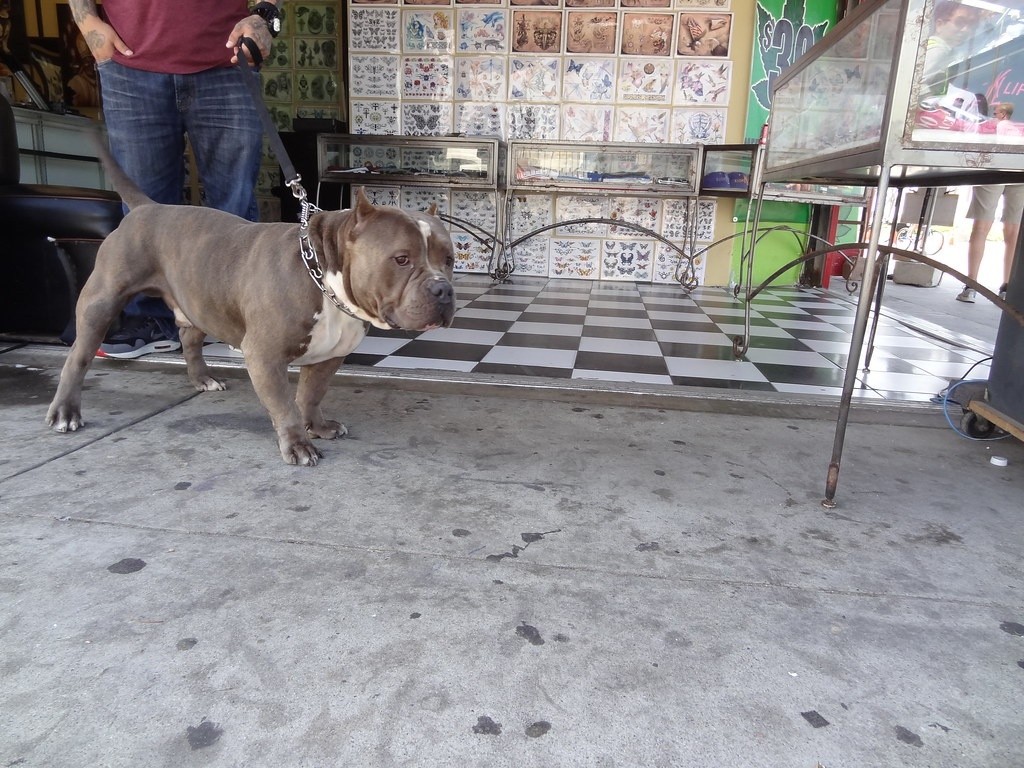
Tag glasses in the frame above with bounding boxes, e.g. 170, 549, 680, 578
944, 16, 979, 32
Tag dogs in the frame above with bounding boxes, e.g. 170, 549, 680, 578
46, 131, 454, 465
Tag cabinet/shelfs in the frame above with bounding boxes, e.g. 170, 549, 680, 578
277, 132, 349, 223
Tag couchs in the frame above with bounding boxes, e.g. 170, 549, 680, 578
0, 93, 125, 334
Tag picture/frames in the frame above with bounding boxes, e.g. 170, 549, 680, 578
699, 144, 758, 199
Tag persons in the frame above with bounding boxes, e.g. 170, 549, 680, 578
68, 0, 277, 358
916, 0, 980, 115
950, 93, 1021, 135
956, 185, 1024, 303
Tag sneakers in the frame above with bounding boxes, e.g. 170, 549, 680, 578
956, 286, 976, 303
97, 316, 181, 358
999, 283, 1008, 300
